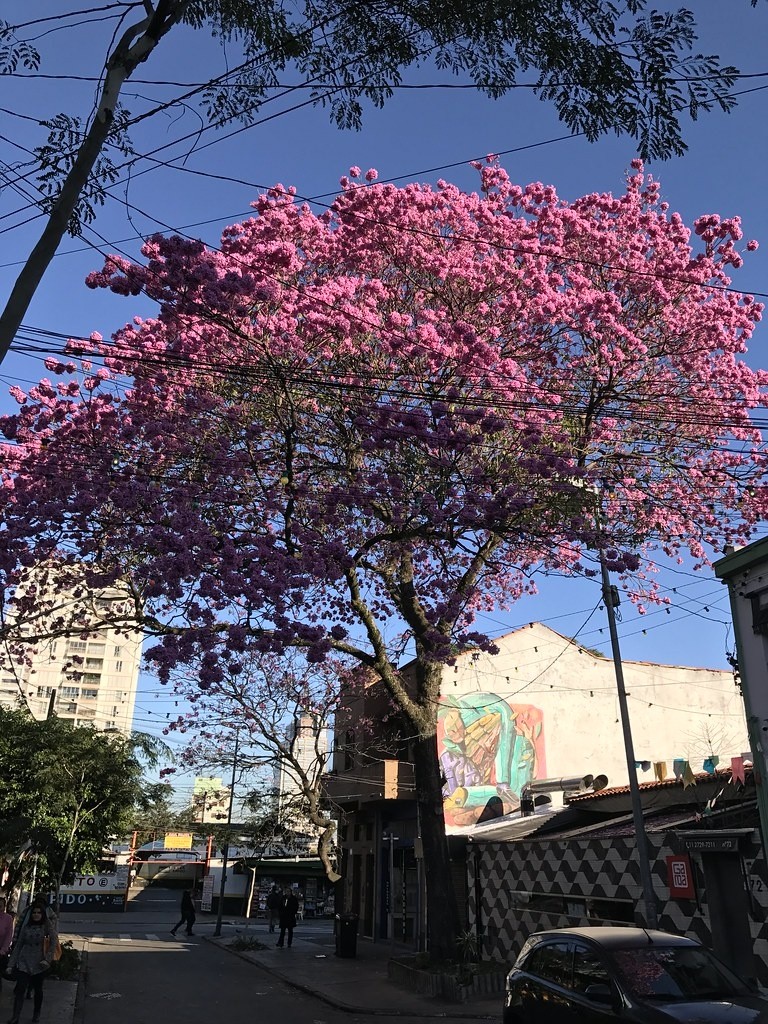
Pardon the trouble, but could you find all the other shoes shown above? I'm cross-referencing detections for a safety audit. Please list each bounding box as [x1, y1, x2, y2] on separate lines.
[275, 943, 292, 948]
[7, 1017, 19, 1024]
[188, 933, 194, 936]
[32, 1017, 39, 1022]
[169, 930, 176, 936]
[26, 991, 32, 999]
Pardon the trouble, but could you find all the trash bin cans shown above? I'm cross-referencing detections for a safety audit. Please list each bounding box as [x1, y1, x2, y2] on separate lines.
[332, 912, 360, 958]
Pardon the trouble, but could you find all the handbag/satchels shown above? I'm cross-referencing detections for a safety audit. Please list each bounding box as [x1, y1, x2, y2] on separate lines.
[44, 920, 62, 960]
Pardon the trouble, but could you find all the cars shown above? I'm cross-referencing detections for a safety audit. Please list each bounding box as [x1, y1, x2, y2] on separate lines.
[502, 926, 768, 1024]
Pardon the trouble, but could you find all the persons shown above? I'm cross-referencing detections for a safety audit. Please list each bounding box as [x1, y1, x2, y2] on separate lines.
[266, 885, 279, 934]
[170, 889, 196, 936]
[276, 888, 299, 948]
[9, 892, 60, 1000]
[0, 896, 13, 981]
[4, 903, 56, 1024]
